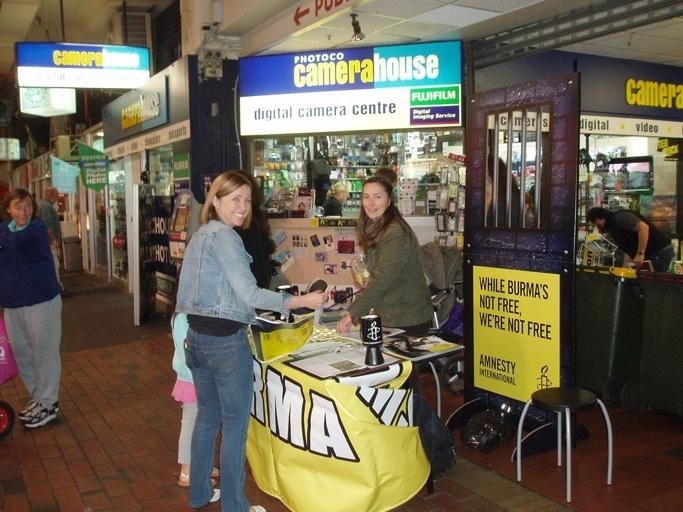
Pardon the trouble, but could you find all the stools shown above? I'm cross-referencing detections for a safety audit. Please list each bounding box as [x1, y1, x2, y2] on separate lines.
[512, 386, 616, 505]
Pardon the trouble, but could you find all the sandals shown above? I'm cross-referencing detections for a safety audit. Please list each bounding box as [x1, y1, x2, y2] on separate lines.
[177, 473, 217, 488]
[211, 468, 220, 478]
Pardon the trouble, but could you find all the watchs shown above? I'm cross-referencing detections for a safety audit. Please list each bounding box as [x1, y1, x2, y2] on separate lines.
[637, 251, 645, 257]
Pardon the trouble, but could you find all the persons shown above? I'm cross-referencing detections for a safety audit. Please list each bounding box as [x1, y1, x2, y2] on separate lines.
[173, 170, 329, 511]
[335, 177, 435, 338]
[34, 184, 75, 299]
[587, 206, 674, 273]
[170, 313, 222, 487]
[1, 187, 66, 428]
[307, 148, 537, 227]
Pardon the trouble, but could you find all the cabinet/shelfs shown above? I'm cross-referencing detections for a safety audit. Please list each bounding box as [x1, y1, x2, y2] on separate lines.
[331, 162, 397, 213]
[253, 161, 308, 216]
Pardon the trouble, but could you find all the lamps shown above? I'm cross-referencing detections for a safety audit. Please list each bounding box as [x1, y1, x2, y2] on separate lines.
[350, 13, 365, 42]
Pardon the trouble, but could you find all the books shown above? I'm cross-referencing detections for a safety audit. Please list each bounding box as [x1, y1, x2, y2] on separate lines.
[381, 333, 461, 361]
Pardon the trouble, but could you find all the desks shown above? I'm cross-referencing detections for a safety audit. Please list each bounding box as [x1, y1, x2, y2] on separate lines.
[245, 314, 463, 512]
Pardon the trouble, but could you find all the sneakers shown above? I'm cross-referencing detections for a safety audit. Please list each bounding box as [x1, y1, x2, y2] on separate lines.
[17, 401, 59, 421]
[25, 407, 57, 428]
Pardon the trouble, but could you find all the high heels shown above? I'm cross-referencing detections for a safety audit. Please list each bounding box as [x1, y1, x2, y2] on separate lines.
[251, 506, 266, 512]
[192, 488, 220, 512]
